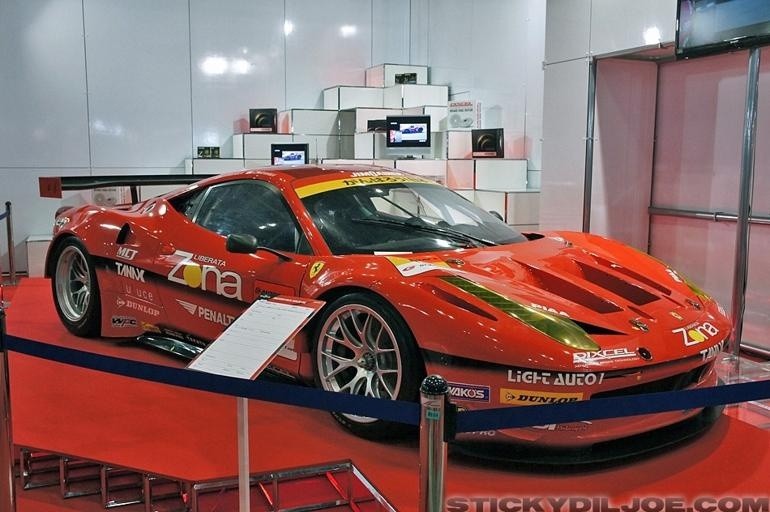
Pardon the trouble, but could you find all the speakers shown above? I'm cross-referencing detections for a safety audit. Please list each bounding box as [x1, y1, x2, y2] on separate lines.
[471, 128, 504, 158]
[249, 108, 277, 133]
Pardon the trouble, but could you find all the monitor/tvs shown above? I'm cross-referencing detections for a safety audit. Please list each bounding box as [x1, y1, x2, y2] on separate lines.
[271, 144, 309, 165]
[675, 0, 770, 62]
[386, 117, 430, 147]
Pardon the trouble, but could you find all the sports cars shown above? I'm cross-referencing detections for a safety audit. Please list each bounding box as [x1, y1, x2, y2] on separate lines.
[43, 165, 733, 468]
